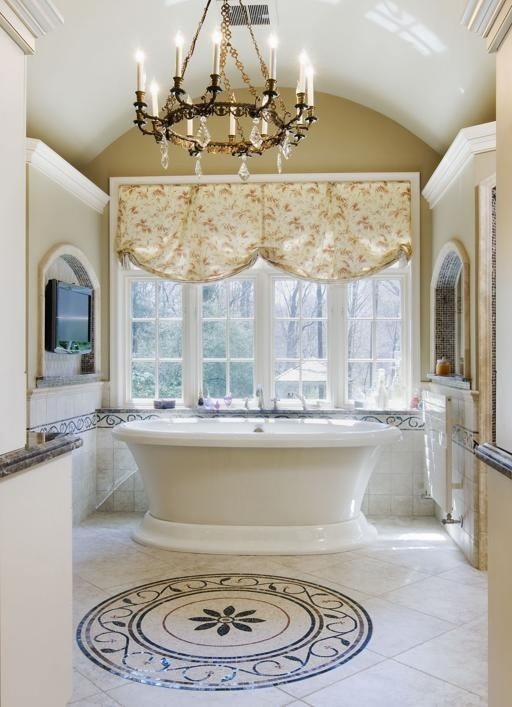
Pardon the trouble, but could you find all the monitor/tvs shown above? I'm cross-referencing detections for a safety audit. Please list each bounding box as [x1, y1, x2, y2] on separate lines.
[45, 278, 94, 355]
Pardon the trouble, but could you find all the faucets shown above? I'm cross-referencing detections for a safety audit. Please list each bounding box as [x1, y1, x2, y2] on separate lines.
[256, 384, 267, 411]
[287, 390, 308, 410]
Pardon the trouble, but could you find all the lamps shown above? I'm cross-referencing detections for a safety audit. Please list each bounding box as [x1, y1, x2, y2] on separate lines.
[133, 0, 318, 181]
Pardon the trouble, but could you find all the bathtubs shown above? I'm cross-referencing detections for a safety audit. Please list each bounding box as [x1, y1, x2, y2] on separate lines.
[111, 418, 402, 526]
